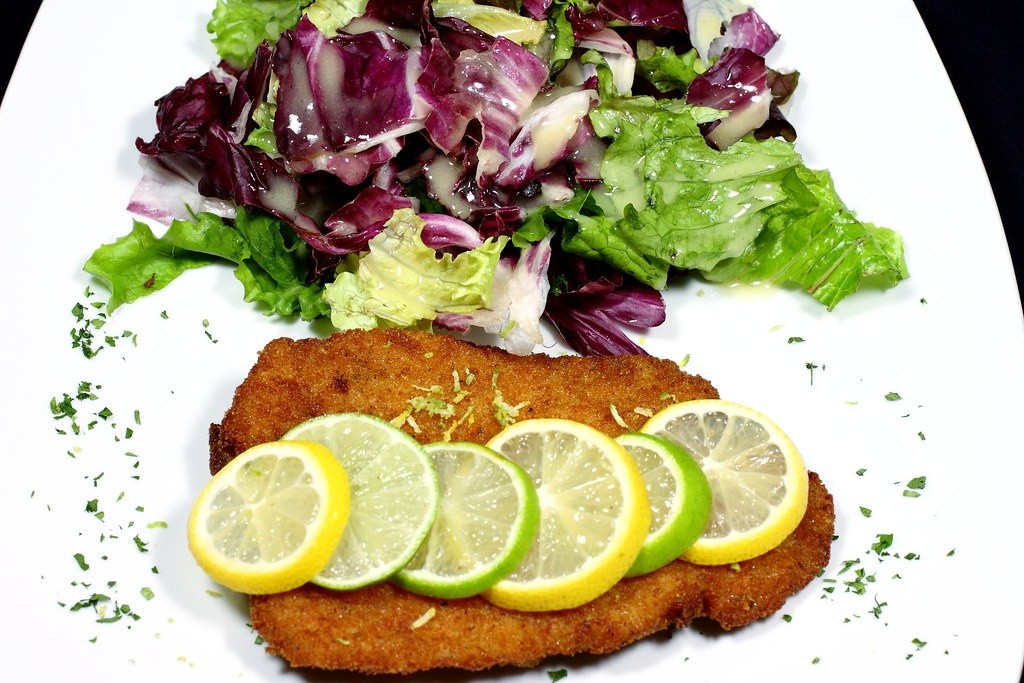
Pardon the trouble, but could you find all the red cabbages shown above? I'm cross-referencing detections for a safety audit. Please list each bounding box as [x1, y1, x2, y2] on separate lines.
[127, 0, 781, 357]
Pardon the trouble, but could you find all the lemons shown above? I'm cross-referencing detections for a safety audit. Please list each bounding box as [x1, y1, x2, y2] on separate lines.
[186, 399, 809, 614]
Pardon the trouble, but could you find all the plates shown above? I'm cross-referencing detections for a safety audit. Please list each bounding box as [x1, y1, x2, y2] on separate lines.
[0, 0, 1024, 683]
